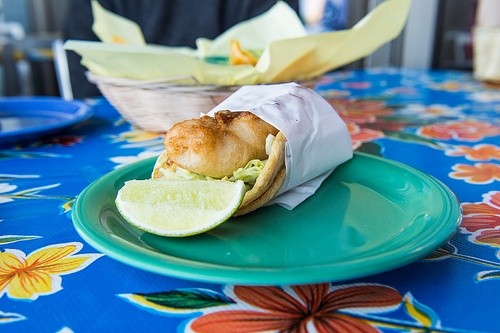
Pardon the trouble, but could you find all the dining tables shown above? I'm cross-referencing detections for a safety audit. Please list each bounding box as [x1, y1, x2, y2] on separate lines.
[0, 67, 500, 332]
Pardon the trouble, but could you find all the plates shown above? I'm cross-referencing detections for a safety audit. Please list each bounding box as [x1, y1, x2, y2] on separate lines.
[0, 97, 93, 142]
[72, 152, 463, 286]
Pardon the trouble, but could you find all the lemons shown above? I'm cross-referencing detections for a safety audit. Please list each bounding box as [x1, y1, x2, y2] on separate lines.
[115, 179, 246, 238]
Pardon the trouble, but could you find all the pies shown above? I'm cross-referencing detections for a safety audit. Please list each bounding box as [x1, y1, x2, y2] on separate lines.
[151, 110, 286, 216]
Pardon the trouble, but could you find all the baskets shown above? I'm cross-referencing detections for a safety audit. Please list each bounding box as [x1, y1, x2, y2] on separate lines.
[85, 67, 321, 133]
